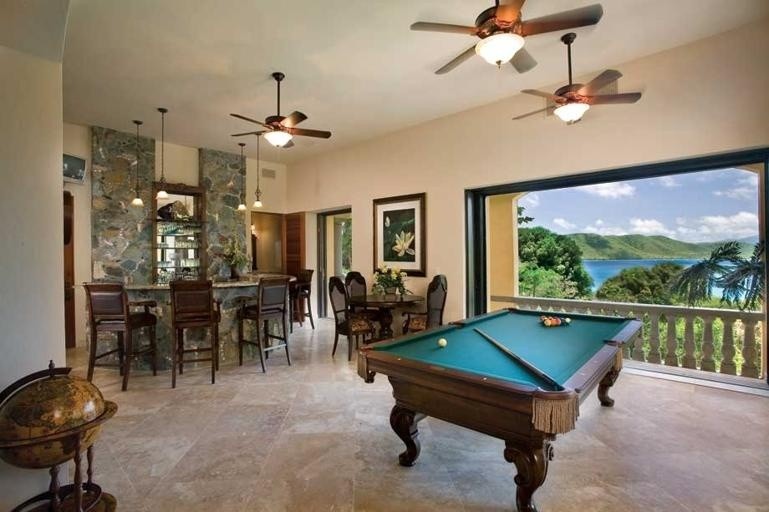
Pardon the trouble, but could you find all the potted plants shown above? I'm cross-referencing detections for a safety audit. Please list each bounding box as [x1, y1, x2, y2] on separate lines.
[216, 223, 253, 280]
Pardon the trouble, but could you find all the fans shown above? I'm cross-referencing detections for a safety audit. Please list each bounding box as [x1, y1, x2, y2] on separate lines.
[410, 0, 603, 75]
[229, 72, 332, 149]
[512, 33, 641, 121]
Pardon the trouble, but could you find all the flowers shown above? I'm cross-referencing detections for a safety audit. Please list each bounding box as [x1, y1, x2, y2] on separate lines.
[372, 262, 413, 296]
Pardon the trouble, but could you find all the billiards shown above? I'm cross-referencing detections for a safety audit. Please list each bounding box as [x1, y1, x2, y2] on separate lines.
[438, 338, 447, 347]
[541, 315, 571, 326]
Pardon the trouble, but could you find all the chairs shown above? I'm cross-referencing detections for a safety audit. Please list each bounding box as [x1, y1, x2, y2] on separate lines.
[81, 283, 157, 391]
[233, 276, 291, 374]
[329, 272, 448, 361]
[168, 279, 221, 388]
[289, 269, 315, 333]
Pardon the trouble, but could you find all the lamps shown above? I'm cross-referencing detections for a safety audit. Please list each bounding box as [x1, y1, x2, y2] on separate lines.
[553, 103, 590, 125]
[156, 107, 169, 199]
[475, 33, 525, 72]
[263, 131, 293, 148]
[237, 141, 246, 211]
[252, 132, 264, 208]
[130, 119, 143, 208]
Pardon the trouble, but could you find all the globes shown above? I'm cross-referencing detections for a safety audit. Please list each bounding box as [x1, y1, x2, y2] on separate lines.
[0, 360, 117, 512]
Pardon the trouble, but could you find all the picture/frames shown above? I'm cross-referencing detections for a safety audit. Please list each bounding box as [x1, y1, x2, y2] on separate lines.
[373, 192, 426, 277]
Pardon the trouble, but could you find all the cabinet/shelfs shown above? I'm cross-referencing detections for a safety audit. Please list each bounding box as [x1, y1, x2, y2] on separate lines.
[155, 218, 202, 286]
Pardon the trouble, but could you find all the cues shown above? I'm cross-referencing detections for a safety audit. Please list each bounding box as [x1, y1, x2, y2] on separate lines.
[474, 327, 558, 387]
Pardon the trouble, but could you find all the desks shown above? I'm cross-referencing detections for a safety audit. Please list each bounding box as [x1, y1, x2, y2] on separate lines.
[357, 306, 645, 512]
